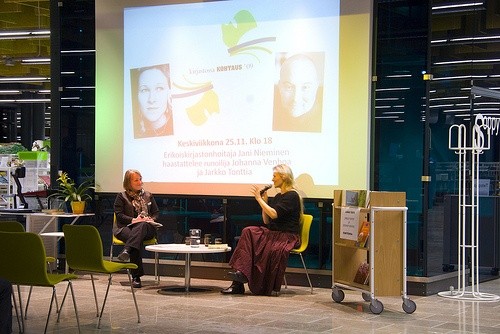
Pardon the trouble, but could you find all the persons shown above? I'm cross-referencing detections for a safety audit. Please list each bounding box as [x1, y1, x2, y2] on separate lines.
[272, 54, 322, 130]
[113, 169, 158, 288]
[221, 165, 304, 295]
[135, 68, 170, 137]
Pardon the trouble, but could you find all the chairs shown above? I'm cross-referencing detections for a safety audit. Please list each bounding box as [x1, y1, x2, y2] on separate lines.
[0, 213, 160, 334]
[284, 214, 314, 293]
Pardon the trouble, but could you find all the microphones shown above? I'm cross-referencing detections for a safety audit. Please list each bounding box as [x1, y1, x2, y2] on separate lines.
[259, 184, 273, 195]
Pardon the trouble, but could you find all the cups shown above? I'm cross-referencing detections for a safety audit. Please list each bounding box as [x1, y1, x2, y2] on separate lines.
[189, 229, 201, 248]
[185, 237, 190, 245]
[204, 234, 211, 246]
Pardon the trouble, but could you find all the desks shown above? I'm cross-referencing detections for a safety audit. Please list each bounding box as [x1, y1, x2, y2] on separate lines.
[145, 244, 232, 295]
[0, 212, 95, 270]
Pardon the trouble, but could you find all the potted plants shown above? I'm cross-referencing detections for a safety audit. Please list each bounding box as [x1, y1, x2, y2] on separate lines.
[46, 171, 96, 214]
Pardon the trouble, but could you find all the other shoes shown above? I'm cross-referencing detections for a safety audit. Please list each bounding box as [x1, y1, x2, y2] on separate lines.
[220, 286, 245, 295]
[227, 271, 248, 283]
[132, 277, 141, 288]
[118, 249, 130, 260]
